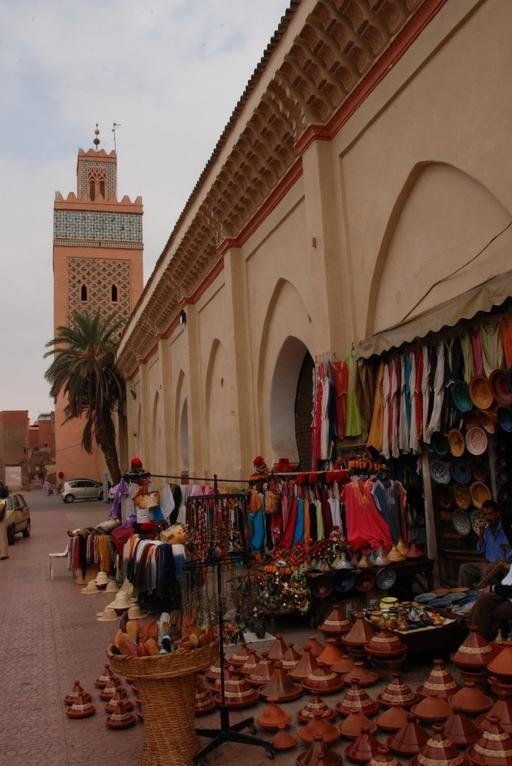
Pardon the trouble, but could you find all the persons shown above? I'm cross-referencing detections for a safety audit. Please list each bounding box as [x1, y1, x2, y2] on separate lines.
[458, 500, 512, 590]
[0, 480, 9, 560]
[249, 455, 272, 494]
[131, 458, 153, 493]
[482, 560, 512, 599]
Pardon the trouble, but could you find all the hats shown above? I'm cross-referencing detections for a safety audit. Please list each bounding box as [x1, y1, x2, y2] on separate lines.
[79, 570, 119, 595]
[95, 590, 148, 623]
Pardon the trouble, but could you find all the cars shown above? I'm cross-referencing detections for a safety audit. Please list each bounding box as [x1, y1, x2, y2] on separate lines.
[60, 477, 104, 503]
[5, 493, 31, 545]
